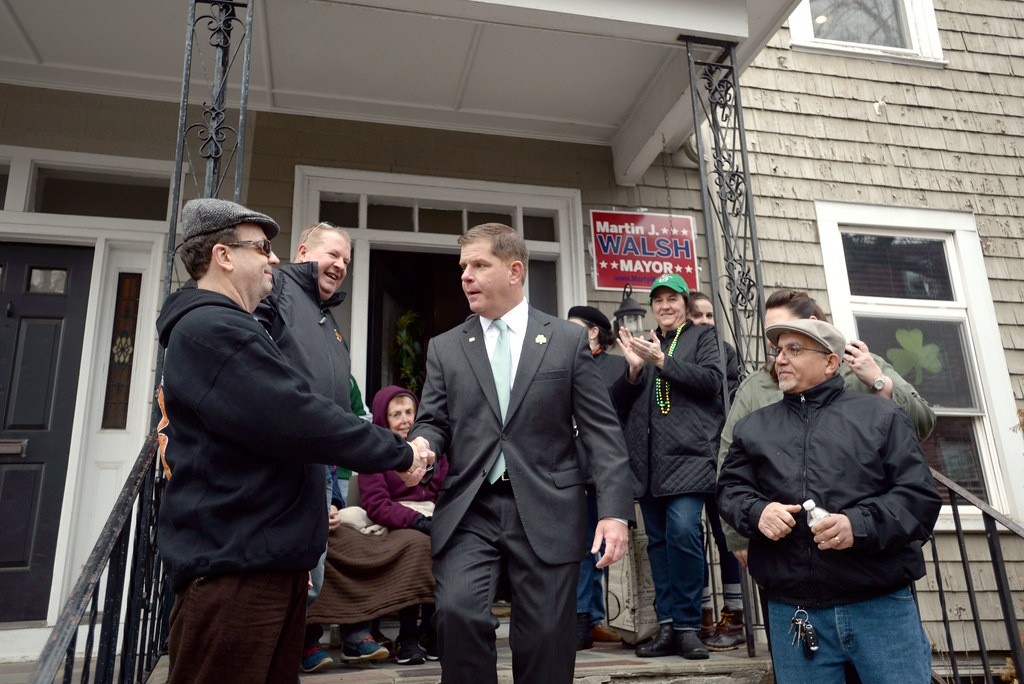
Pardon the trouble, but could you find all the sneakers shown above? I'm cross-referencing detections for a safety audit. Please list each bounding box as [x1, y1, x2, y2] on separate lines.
[394, 636, 426, 665]
[301, 644, 333, 672]
[417, 631, 440, 661]
[340, 636, 389, 661]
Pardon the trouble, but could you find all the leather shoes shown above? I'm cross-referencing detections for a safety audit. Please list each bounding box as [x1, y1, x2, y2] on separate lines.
[590, 624, 621, 642]
[634, 624, 676, 657]
[676, 630, 710, 659]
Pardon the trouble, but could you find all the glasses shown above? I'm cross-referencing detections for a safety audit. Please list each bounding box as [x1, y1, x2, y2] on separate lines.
[772, 345, 831, 358]
[389, 410, 415, 419]
[303, 222, 333, 244]
[222, 240, 272, 257]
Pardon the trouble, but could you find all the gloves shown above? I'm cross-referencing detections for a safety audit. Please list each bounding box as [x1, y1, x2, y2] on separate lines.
[412, 513, 434, 536]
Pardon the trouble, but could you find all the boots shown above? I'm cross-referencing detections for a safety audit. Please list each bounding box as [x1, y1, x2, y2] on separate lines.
[575, 612, 592, 651]
[704, 605, 747, 652]
[698, 607, 717, 642]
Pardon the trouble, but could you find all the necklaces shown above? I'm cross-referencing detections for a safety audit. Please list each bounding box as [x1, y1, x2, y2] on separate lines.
[654, 321, 687, 414]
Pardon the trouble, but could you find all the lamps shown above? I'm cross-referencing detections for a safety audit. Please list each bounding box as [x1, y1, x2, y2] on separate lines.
[611, 283, 646, 337]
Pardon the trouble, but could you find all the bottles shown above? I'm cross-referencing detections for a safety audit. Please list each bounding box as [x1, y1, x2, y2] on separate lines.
[803, 499, 831, 544]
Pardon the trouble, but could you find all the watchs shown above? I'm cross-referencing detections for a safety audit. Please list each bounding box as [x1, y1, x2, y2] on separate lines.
[867, 374, 887, 392]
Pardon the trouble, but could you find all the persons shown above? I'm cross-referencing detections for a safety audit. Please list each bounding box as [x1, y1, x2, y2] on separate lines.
[715, 318, 943, 684]
[717, 288, 936, 571]
[398, 224, 629, 683]
[298, 465, 388, 673]
[252, 219, 367, 597]
[565, 306, 632, 648]
[684, 289, 746, 651]
[606, 271, 726, 658]
[355, 383, 440, 665]
[153, 198, 433, 684]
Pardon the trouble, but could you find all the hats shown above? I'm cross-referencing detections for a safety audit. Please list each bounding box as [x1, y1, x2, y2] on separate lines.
[649, 274, 689, 296]
[568, 306, 612, 332]
[765, 319, 846, 367]
[183, 198, 281, 242]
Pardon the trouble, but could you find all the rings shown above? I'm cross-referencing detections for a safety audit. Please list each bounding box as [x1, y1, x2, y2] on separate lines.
[835, 536, 841, 544]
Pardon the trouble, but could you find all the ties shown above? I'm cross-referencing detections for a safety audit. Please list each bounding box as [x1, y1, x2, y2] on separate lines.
[485, 319, 511, 485]
